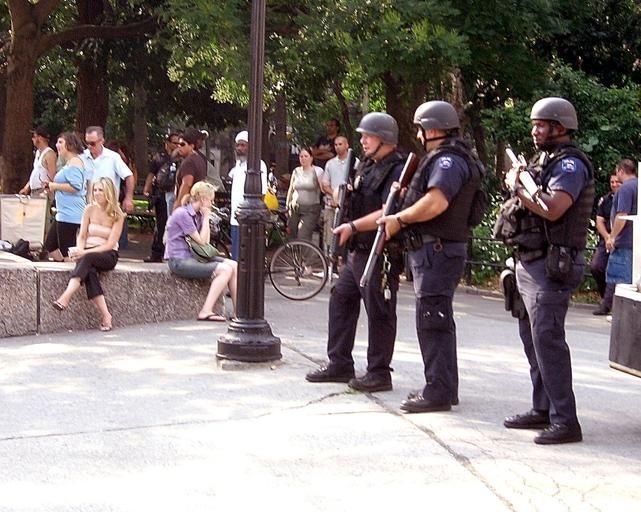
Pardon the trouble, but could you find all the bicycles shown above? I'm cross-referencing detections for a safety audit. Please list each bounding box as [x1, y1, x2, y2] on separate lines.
[208, 205, 328, 300]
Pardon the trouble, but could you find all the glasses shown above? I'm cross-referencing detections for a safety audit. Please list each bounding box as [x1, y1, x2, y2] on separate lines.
[169, 141, 178, 144]
[86, 140, 101, 146]
[180, 143, 186, 146]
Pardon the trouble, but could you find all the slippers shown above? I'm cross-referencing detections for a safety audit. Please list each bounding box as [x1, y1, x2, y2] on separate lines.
[198, 313, 225, 321]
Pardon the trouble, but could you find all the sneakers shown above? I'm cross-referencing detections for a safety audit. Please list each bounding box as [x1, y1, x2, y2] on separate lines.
[143, 256, 163, 262]
[535, 422, 581, 444]
[348, 371, 392, 391]
[52, 300, 66, 311]
[592, 305, 609, 313]
[504, 410, 550, 428]
[401, 392, 458, 410]
[100, 324, 111, 331]
[305, 365, 355, 382]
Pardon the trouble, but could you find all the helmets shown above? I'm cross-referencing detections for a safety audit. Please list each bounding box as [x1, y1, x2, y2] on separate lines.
[356, 112, 399, 145]
[414, 101, 460, 130]
[530, 97, 578, 130]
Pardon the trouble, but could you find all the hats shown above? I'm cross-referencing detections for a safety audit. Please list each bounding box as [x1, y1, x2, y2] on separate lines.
[29, 127, 51, 138]
[235, 131, 249, 143]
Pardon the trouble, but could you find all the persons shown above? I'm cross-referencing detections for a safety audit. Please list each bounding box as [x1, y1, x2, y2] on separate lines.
[493, 98, 595, 445]
[286, 117, 361, 276]
[306, 112, 407, 393]
[19, 127, 138, 262]
[51, 177, 126, 331]
[376, 101, 479, 413]
[163, 182, 237, 322]
[590, 159, 638, 314]
[142, 128, 267, 282]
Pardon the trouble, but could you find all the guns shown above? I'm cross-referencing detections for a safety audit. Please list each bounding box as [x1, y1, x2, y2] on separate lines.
[360, 152, 421, 289]
[328, 148, 357, 286]
[505, 147, 549, 212]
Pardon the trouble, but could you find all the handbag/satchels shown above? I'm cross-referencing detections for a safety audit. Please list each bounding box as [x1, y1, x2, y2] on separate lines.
[271, 224, 297, 242]
[14, 239, 29, 257]
[186, 235, 218, 263]
[264, 189, 278, 210]
